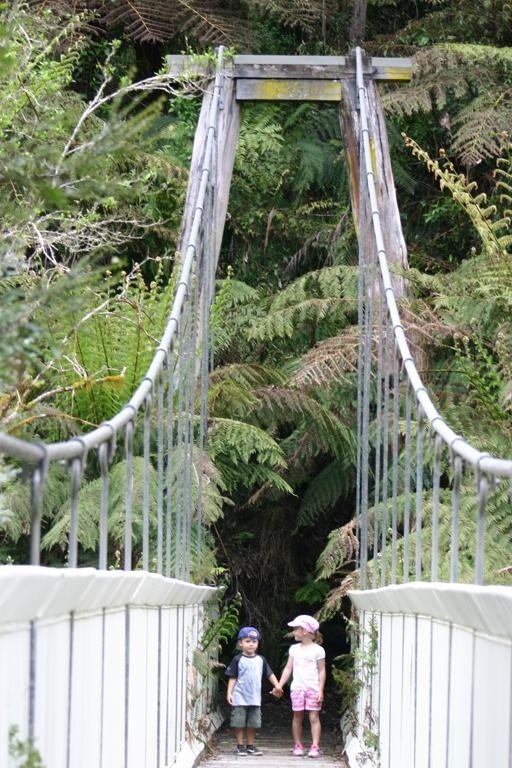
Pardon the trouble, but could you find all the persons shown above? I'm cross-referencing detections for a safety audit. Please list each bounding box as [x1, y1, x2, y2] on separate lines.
[224, 615, 326, 759]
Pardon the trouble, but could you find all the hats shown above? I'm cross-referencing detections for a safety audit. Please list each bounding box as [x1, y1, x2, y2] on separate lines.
[237, 626, 260, 641]
[286, 614, 320, 634]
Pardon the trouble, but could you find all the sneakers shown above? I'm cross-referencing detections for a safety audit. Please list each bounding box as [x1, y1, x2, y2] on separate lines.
[307, 744, 322, 758]
[235, 745, 264, 757]
[293, 742, 305, 757]
